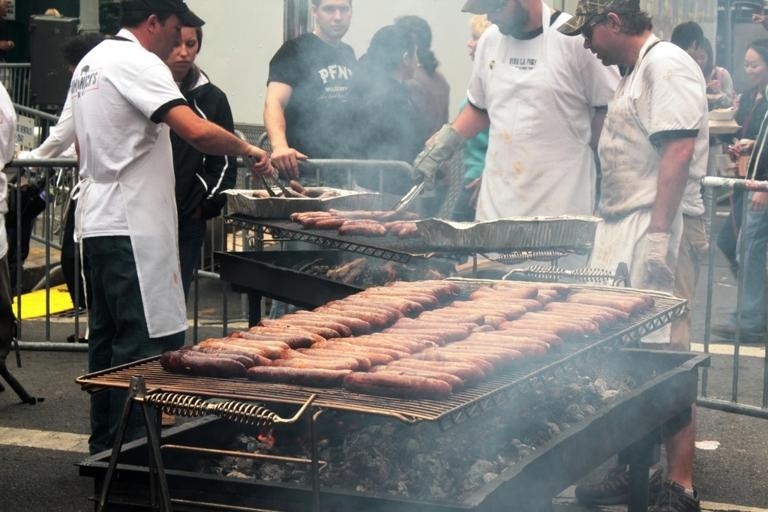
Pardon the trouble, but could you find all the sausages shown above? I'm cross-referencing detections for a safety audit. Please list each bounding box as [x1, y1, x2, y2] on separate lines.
[248, 180, 342, 199]
[342, 289, 654, 395]
[245, 282, 568, 386]
[289, 208, 424, 238]
[161, 279, 461, 375]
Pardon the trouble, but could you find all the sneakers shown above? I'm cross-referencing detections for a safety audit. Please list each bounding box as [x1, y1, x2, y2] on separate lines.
[574, 463, 664, 505]
[648, 478, 702, 512]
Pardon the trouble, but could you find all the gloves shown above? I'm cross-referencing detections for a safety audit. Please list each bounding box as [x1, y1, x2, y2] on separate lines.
[644, 232, 674, 283]
[411, 123, 466, 195]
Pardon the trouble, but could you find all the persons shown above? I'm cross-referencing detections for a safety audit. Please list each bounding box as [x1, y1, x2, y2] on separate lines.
[670, 11, 767, 343]
[393, 14, 449, 142]
[346, 23, 426, 197]
[163, 25, 238, 305]
[262, 0, 357, 319]
[449, 12, 494, 222]
[554, 0, 710, 512]
[409, 0, 623, 274]
[0, 0, 126, 393]
[68, 0, 274, 458]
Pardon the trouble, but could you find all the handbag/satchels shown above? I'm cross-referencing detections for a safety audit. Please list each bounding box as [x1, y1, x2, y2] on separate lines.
[738, 154, 752, 177]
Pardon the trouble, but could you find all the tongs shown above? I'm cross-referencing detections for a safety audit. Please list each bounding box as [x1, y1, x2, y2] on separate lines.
[95, 373, 173, 511]
[390, 173, 425, 214]
[241, 152, 295, 198]
[612, 261, 632, 288]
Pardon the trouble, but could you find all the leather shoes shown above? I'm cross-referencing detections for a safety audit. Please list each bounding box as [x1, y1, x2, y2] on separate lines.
[706, 321, 766, 344]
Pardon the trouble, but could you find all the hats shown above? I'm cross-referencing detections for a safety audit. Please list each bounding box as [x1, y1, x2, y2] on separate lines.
[556, 0, 639, 36]
[119, 0, 206, 27]
[461, 0, 507, 14]
